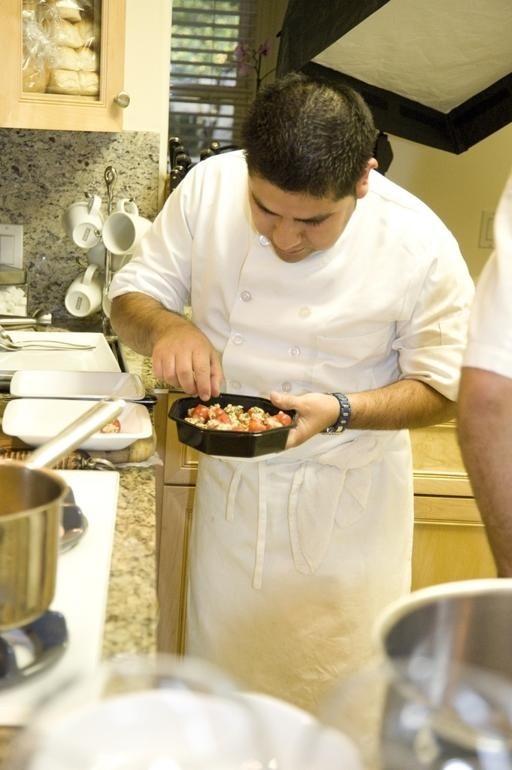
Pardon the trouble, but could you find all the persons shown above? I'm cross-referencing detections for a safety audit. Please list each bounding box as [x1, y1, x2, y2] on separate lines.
[453, 172, 511, 580]
[105, 72, 474, 769]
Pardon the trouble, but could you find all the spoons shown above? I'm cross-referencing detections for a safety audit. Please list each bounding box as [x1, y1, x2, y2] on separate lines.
[0, 326, 96, 352]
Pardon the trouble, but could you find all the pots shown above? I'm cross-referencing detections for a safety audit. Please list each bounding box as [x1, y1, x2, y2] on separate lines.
[376, 574, 512, 770]
[0, 453, 89, 688]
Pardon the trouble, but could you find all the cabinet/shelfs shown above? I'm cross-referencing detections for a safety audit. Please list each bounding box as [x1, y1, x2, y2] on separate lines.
[0, 0, 131, 135]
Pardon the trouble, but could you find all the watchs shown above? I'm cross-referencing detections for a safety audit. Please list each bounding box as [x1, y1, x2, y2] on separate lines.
[320, 392, 352, 435]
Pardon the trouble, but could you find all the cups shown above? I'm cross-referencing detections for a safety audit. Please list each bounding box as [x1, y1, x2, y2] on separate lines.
[305, 652, 512, 770]
[19, 648, 273, 770]
[105, 198, 154, 255]
[64, 265, 104, 316]
[87, 244, 127, 270]
[58, 196, 103, 249]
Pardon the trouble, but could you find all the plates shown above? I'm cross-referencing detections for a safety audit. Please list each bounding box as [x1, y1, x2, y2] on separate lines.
[4, 397, 153, 453]
[8, 367, 146, 401]
[169, 390, 304, 455]
